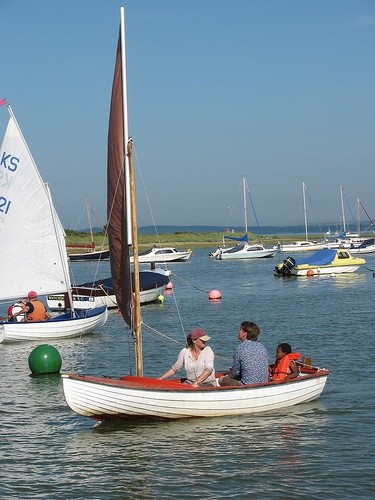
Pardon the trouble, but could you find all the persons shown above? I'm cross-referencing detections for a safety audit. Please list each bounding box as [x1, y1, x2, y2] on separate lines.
[219, 321, 269, 387]
[217, 246, 222, 254]
[8, 291, 51, 323]
[268, 343, 299, 382]
[157, 328, 217, 389]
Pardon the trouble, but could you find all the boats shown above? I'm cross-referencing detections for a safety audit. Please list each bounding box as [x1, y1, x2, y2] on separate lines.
[49, 262, 170, 304]
[209, 246, 231, 258]
[276, 247, 366, 276]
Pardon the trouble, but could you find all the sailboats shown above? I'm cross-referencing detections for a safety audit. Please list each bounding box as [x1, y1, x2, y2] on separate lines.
[324, 186, 360, 238]
[59, 8, 327, 419]
[324, 198, 375, 253]
[217, 177, 279, 259]
[273, 181, 326, 251]
[0, 104, 109, 343]
[131, 246, 193, 263]
[69, 194, 109, 259]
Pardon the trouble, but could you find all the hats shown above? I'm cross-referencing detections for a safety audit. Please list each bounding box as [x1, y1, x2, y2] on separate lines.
[28, 291, 37, 300]
[20, 298, 27, 305]
[190, 328, 211, 342]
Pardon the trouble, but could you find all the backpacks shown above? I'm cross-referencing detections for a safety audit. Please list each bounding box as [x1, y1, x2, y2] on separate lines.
[8, 304, 23, 322]
